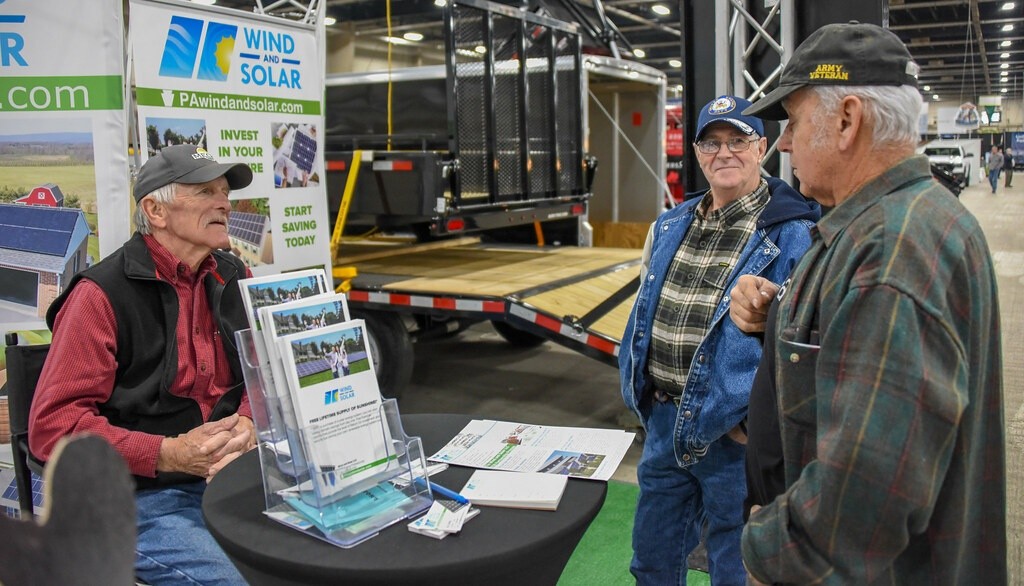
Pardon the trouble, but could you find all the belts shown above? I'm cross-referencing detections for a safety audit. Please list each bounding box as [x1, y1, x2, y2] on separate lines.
[650, 385, 674, 403]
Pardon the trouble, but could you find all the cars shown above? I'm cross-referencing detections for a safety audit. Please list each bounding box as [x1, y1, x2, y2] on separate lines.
[1013, 139, 1024, 170]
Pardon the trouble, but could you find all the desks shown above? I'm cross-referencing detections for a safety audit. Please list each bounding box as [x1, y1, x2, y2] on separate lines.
[201, 414, 608, 586]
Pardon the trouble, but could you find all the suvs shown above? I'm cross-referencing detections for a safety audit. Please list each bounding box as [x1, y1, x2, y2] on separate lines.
[922, 143, 973, 190]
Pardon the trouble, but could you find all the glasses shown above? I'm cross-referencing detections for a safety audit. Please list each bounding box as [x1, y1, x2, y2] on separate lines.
[694, 136, 757, 155]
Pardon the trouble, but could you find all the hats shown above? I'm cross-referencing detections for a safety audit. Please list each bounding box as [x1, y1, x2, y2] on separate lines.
[740, 20, 921, 121]
[695, 95, 764, 142]
[134, 144, 254, 205]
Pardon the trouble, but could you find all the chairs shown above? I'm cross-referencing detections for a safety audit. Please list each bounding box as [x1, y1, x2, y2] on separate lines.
[5, 332, 46, 519]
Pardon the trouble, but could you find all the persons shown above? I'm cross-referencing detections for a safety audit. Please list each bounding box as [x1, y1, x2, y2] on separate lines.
[28, 144, 268, 586]
[279, 284, 302, 303]
[618, 93, 821, 586]
[323, 338, 350, 378]
[303, 309, 326, 329]
[729, 20, 1015, 586]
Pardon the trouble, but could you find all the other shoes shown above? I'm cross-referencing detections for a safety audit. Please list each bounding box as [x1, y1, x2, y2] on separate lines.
[992, 190, 995, 194]
[1005, 185, 1013, 187]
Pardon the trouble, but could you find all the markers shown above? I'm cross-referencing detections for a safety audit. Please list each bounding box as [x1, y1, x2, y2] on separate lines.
[415, 477, 468, 505]
